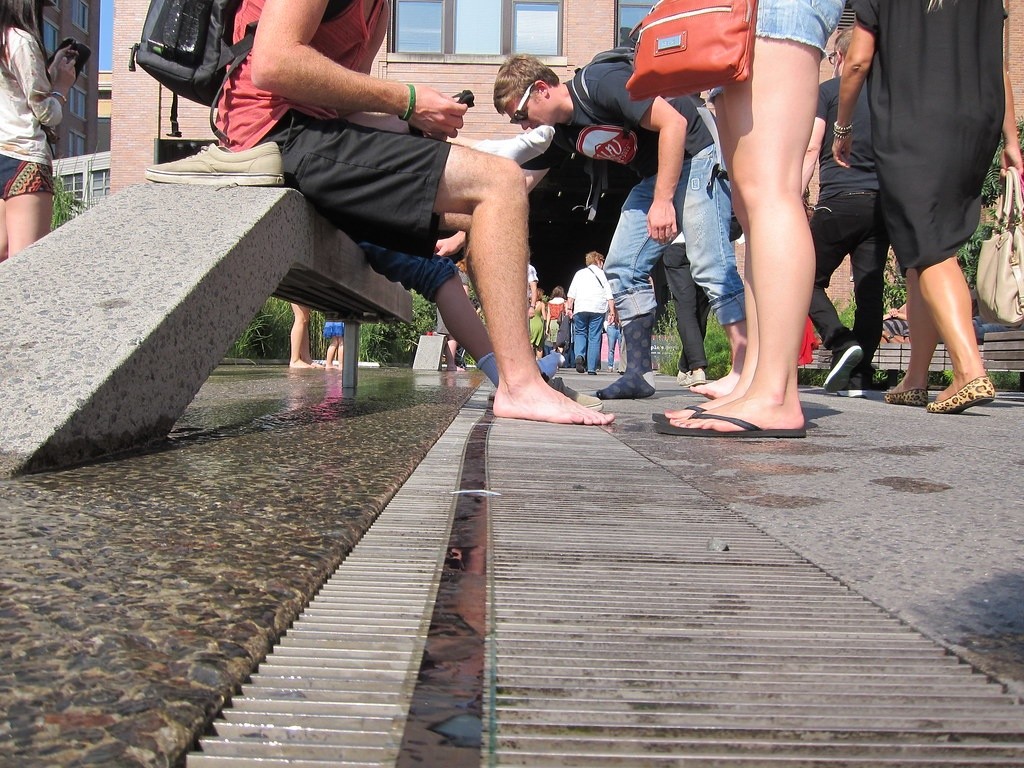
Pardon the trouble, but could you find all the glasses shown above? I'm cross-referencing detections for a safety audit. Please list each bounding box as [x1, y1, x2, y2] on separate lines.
[829, 52, 838, 65]
[509, 82, 537, 124]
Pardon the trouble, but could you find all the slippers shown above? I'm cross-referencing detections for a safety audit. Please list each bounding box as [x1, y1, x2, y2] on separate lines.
[654, 414, 806, 438]
[652, 406, 708, 422]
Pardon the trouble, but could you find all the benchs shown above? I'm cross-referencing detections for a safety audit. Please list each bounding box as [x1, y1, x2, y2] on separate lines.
[0, 179, 447, 481]
[798, 332, 1024, 387]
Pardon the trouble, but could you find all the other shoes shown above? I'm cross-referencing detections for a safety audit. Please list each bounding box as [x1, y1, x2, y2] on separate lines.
[607, 368, 613, 372]
[620, 372, 624, 374]
[848, 383, 871, 398]
[822, 345, 864, 392]
[589, 372, 596, 375]
[884, 389, 928, 406]
[576, 355, 585, 373]
[926, 376, 995, 415]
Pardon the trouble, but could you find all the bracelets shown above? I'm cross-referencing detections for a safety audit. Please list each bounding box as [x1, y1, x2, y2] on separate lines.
[530, 306, 535, 309]
[399, 83, 415, 122]
[610, 314, 615, 317]
[567, 307, 572, 310]
[52, 91, 67, 103]
[832, 120, 853, 139]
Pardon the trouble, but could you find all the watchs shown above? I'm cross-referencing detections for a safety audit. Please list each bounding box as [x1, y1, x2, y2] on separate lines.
[887, 334, 894, 341]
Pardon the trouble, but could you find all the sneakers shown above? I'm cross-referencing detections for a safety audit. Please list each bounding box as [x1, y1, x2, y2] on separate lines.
[548, 377, 603, 412]
[679, 368, 707, 387]
[676, 371, 692, 384]
[145, 141, 285, 187]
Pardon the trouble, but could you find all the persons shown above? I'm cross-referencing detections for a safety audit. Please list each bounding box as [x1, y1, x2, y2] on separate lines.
[0, 0, 79, 262]
[434, 47, 750, 400]
[650, 1, 845, 437]
[830, 0, 1024, 413]
[289, 301, 323, 370]
[323, 322, 344, 370]
[800, 24, 890, 398]
[879, 303, 1020, 344]
[352, 124, 564, 389]
[217, 0, 615, 425]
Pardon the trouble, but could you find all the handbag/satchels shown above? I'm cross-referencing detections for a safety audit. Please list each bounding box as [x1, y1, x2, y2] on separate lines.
[625, 0, 758, 100]
[976, 166, 1024, 327]
[556, 315, 571, 355]
[557, 301, 565, 326]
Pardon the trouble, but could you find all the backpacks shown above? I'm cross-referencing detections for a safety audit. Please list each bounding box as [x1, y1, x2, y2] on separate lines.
[129, 0, 355, 148]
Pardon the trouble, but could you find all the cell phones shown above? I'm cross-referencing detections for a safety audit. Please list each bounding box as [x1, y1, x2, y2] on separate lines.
[65, 50, 79, 64]
